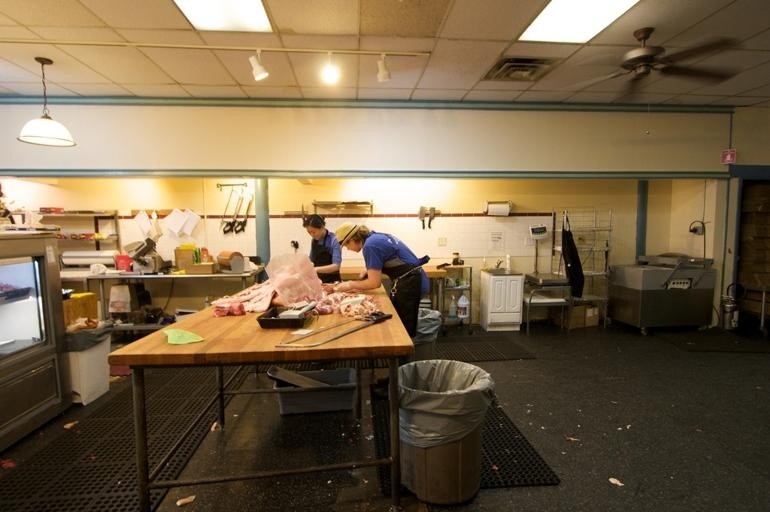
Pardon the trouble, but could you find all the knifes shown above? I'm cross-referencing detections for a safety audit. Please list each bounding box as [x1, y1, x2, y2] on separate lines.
[419, 207, 425, 229]
[428, 208, 435, 228]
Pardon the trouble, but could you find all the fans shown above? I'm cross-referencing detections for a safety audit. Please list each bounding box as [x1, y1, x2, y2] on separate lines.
[556, 24, 742, 99]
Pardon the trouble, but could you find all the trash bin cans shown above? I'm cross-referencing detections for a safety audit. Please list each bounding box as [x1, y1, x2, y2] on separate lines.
[412, 308, 441, 360]
[65, 320, 115, 405]
[398, 360, 495, 504]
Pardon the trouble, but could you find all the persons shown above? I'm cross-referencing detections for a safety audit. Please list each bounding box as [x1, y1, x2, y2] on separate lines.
[302, 214, 342, 283]
[331, 221, 431, 366]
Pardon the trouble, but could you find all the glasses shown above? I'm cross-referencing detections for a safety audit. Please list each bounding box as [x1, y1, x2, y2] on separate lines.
[303, 216, 318, 221]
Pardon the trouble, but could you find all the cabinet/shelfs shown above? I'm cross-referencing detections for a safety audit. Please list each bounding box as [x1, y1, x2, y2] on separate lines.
[8, 210, 120, 298]
[479, 269, 524, 331]
[552, 206, 613, 333]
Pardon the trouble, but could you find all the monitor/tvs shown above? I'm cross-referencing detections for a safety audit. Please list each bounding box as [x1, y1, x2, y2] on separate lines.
[0, 251, 52, 365]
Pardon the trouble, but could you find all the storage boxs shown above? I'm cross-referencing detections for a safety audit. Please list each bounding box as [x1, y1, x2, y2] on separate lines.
[564, 305, 599, 330]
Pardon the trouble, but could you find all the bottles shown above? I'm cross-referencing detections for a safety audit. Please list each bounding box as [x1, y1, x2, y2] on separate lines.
[449, 294, 457, 316]
[453, 253, 459, 265]
[194, 248, 200, 263]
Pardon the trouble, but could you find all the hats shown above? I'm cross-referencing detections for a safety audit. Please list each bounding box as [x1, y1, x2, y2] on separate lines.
[335, 223, 360, 247]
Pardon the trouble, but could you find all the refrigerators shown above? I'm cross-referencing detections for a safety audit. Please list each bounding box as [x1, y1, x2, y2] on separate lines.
[0, 231, 74, 451]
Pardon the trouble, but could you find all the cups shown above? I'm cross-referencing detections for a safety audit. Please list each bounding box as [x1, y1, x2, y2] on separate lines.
[460, 260, 464, 264]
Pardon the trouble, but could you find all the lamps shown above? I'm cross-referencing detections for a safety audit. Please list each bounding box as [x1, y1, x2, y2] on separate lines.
[14, 56, 74, 148]
[375, 53, 393, 82]
[318, 52, 342, 86]
[246, 48, 274, 82]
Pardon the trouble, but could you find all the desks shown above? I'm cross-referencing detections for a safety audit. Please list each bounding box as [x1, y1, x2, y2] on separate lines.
[83, 266, 263, 336]
[340, 265, 446, 313]
[105, 282, 415, 512]
